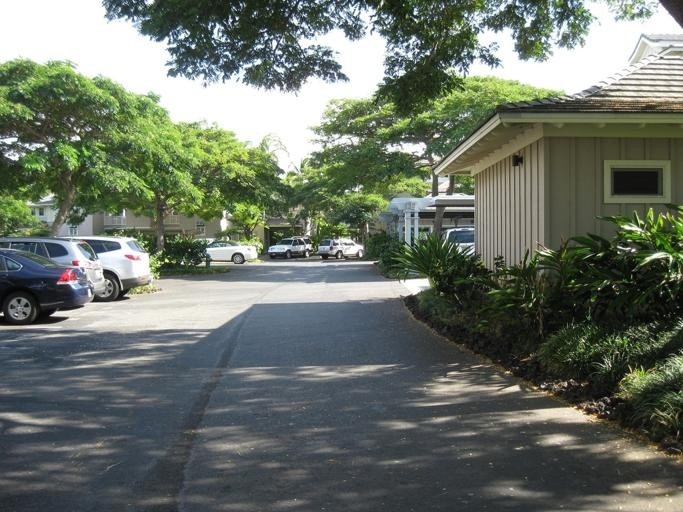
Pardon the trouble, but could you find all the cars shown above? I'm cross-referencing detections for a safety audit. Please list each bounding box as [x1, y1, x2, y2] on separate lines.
[267, 236, 363, 259]
[0, 235, 152, 325]
[191, 241, 257, 265]
[440, 227, 474, 255]
